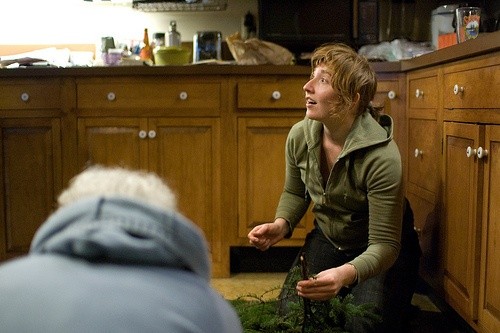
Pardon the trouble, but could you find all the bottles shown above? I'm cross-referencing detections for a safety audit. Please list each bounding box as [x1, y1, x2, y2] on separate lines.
[140, 28, 155, 65]
[241, 10, 256, 40]
[165, 20, 180, 47]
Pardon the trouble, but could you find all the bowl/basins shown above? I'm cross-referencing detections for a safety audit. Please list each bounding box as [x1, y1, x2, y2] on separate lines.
[152, 46, 191, 65]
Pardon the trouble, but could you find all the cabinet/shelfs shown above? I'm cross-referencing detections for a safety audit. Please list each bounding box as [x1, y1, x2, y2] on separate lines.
[0, 49, 500, 333]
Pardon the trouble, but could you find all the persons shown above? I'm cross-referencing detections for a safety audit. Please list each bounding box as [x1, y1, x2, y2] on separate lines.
[0, 165, 243, 333]
[247, 43, 420, 333]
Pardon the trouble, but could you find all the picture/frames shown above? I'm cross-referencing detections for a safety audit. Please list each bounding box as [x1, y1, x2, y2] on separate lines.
[193, 31, 222, 62]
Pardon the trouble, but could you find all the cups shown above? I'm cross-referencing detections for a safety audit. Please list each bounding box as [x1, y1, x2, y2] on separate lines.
[456, 7, 481, 43]
[108, 48, 123, 66]
[102, 37, 115, 66]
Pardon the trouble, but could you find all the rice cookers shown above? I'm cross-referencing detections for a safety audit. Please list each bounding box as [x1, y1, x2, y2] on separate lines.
[431, 4, 460, 50]
